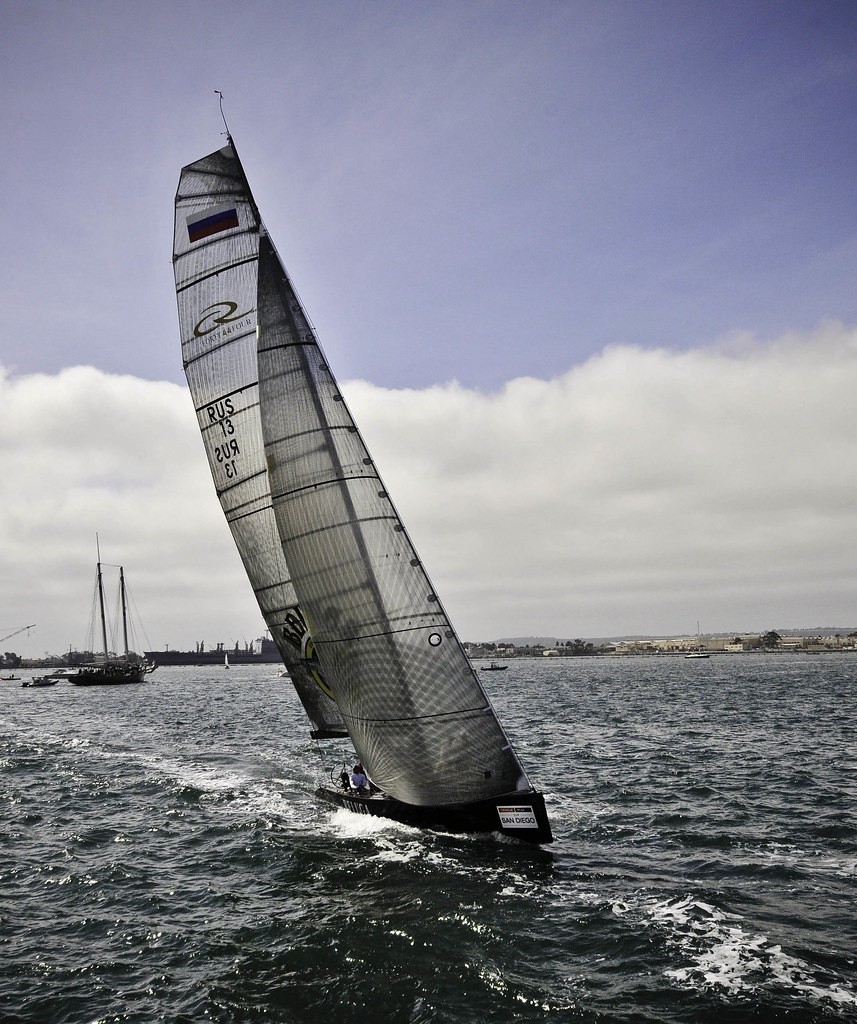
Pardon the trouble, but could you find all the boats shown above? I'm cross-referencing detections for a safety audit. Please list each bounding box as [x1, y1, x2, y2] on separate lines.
[1, 674, 21, 680]
[44, 669, 79, 678]
[278, 666, 290, 677]
[480, 660, 508, 671]
[20, 676, 59, 687]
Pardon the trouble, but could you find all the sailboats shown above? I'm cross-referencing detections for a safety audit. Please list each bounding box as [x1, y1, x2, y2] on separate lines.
[108, 566, 158, 675]
[172, 90, 554, 848]
[225, 653, 230, 669]
[685, 620, 709, 658]
[68, 644, 76, 670]
[67, 532, 144, 686]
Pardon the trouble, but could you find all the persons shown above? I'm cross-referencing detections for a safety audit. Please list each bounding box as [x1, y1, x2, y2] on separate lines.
[79, 667, 127, 676]
[357, 762, 367, 779]
[352, 766, 366, 788]
